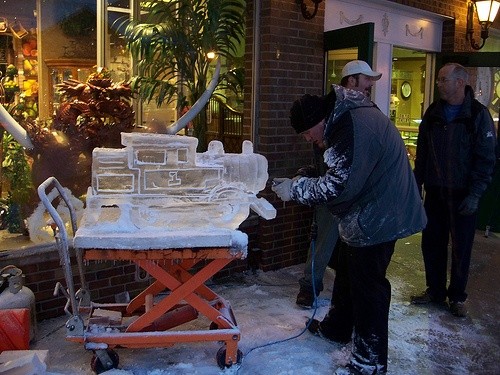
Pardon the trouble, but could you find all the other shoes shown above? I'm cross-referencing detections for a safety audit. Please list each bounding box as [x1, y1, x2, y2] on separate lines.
[296, 288, 315, 308]
[410, 291, 446, 303]
[449, 300, 468, 316]
[306, 318, 321, 336]
[335, 362, 369, 375]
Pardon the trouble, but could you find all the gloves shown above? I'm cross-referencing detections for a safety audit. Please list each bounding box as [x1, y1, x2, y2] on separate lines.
[458, 186, 482, 215]
[414, 174, 424, 200]
[272, 178, 293, 202]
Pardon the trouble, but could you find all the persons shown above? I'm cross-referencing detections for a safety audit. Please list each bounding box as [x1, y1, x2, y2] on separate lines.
[296, 61, 383, 306]
[271, 83, 427, 375]
[410, 62, 497, 317]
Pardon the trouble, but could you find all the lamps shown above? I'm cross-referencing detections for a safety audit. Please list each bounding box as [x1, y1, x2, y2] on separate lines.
[466, 0, 500, 49]
[295, 0, 322, 19]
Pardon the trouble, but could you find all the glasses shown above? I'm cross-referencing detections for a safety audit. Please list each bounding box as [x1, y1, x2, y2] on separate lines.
[436, 78, 457, 84]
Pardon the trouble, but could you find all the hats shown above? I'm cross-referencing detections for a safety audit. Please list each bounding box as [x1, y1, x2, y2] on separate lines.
[290, 94, 331, 134]
[343, 60, 382, 81]
